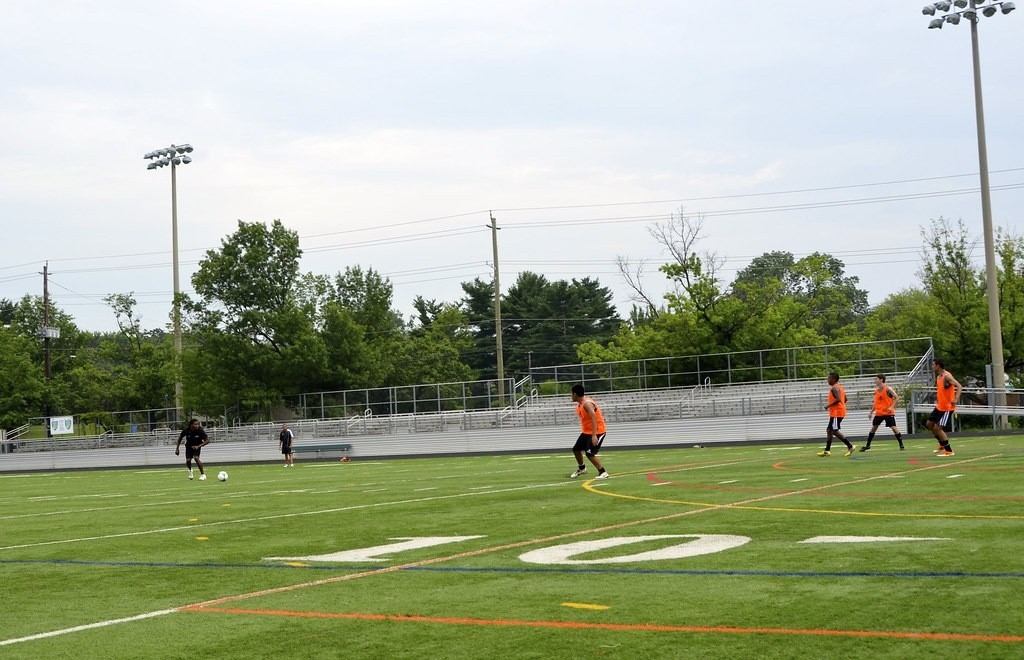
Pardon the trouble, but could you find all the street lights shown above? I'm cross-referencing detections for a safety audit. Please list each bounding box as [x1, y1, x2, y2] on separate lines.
[142, 142, 195, 431]
[920, 0, 1018, 432]
[494, 323, 522, 407]
[524, 351, 535, 396]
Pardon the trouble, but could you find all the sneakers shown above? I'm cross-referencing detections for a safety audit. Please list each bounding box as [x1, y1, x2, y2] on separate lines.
[817, 450, 830, 456]
[595, 472, 609, 479]
[188, 468, 193, 479]
[199, 474, 206, 480]
[933, 445, 944, 453]
[845, 445, 856, 456]
[937, 451, 954, 456]
[571, 468, 587, 478]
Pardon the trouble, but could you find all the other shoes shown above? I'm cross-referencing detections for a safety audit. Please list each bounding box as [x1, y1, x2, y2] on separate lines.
[284, 464, 288, 467]
[899, 445, 904, 450]
[291, 464, 294, 467]
[860, 446, 870, 452]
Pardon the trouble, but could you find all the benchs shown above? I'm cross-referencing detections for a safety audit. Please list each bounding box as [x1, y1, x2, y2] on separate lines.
[13, 373, 932, 463]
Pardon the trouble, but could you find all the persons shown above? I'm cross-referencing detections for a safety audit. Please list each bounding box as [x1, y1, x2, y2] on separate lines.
[175, 418, 210, 481]
[279, 423, 294, 467]
[570, 383, 610, 479]
[816, 372, 856, 457]
[926, 358, 962, 457]
[859, 373, 905, 452]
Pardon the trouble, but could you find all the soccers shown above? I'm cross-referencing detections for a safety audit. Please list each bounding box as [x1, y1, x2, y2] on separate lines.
[217, 471, 228, 482]
